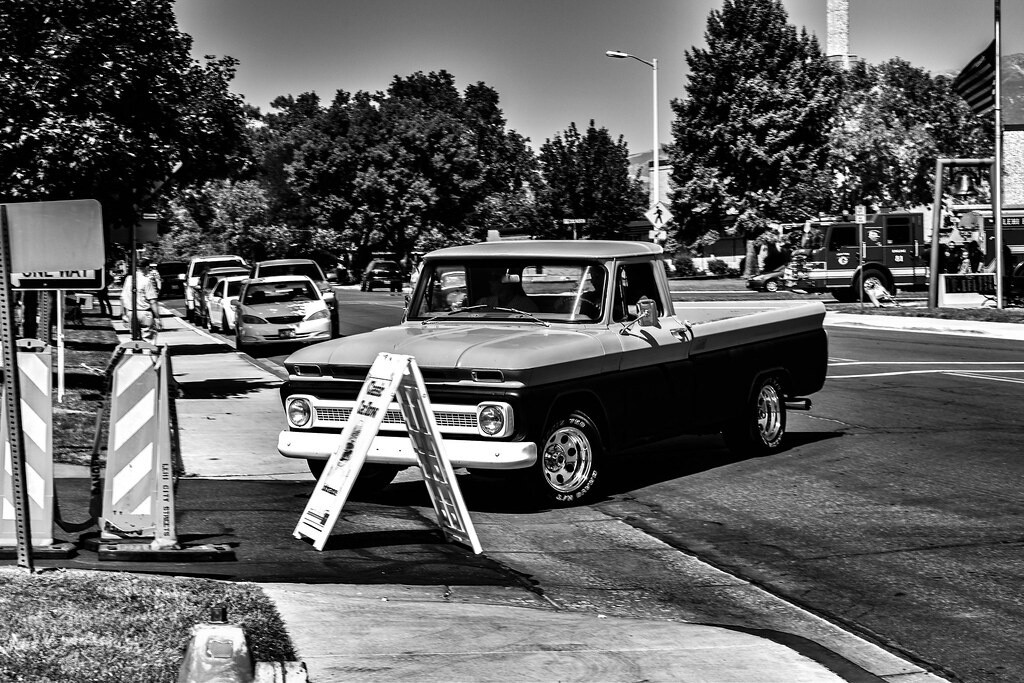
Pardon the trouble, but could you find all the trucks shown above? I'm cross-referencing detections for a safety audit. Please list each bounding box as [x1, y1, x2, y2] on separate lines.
[798, 203, 1024, 302]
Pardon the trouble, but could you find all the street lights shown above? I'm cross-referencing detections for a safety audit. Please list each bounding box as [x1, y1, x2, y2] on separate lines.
[604, 48, 662, 244]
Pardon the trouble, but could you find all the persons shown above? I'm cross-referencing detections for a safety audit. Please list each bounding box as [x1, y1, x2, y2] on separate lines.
[97, 273, 114, 317]
[116, 258, 162, 345]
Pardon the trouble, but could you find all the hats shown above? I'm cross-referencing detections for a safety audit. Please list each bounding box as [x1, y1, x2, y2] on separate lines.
[136, 258, 153, 267]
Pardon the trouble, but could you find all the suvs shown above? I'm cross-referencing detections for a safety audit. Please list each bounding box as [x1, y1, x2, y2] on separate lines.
[257, 259, 339, 340]
[177, 255, 244, 324]
[154, 260, 187, 303]
[190, 266, 250, 329]
[361, 260, 404, 292]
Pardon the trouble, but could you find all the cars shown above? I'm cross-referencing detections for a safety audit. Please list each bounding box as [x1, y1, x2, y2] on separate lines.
[406, 259, 467, 319]
[203, 276, 250, 335]
[231, 273, 335, 355]
[745, 265, 786, 293]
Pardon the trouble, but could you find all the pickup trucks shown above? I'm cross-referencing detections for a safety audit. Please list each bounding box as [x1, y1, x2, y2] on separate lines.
[276, 241, 830, 510]
